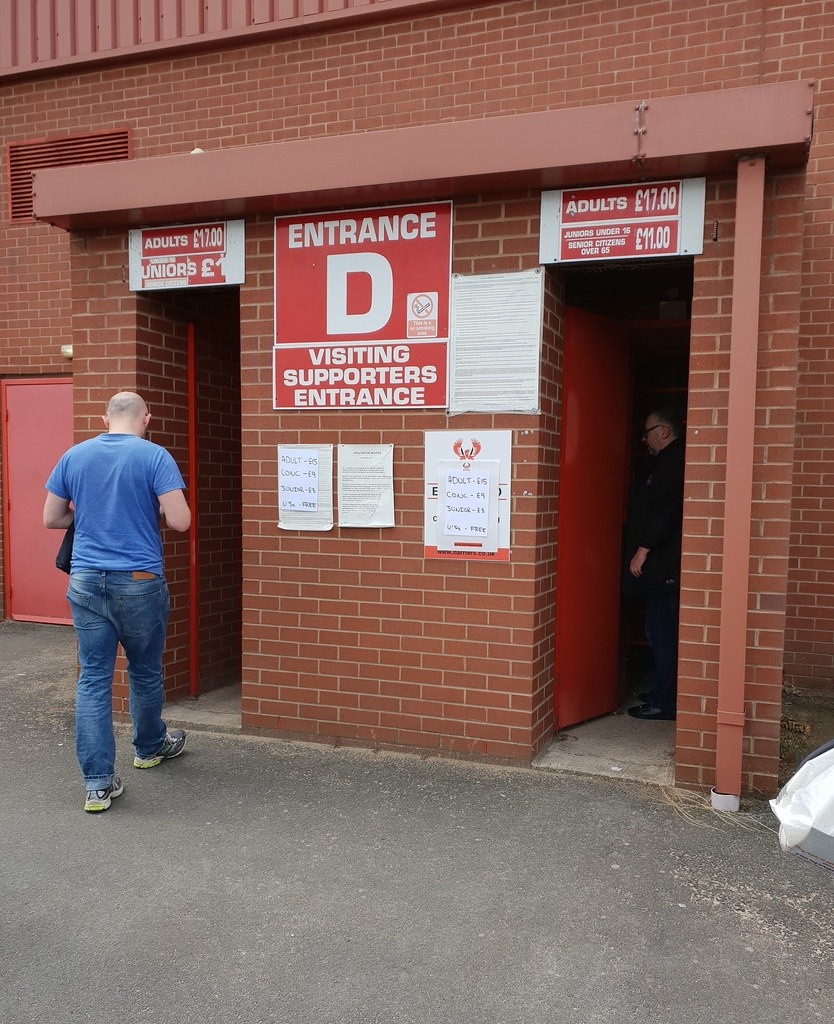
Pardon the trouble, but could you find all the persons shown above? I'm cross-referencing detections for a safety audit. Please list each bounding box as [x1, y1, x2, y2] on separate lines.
[626, 406, 685, 720]
[43, 392, 193, 811]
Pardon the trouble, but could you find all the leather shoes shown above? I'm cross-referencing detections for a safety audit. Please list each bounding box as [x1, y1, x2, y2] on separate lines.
[628, 702, 677, 720]
[636, 693, 653, 701]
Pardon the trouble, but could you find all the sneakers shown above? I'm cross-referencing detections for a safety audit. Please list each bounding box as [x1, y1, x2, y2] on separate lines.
[84, 777, 123, 811]
[133, 730, 186, 768]
